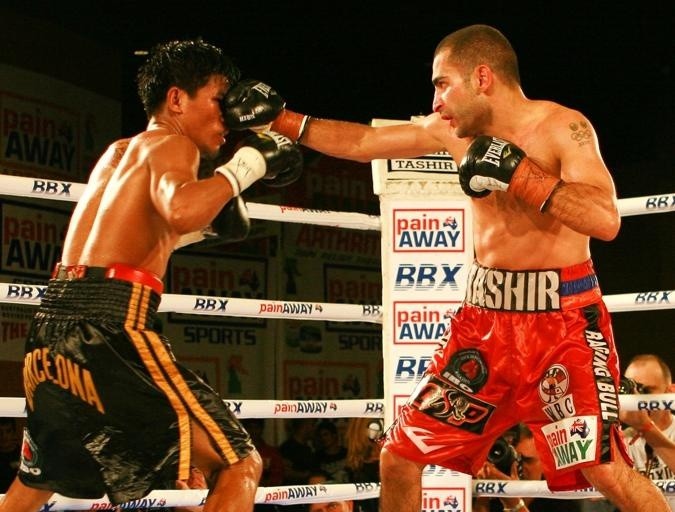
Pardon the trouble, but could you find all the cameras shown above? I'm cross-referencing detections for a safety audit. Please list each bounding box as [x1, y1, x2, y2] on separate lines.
[486, 436, 522, 476]
[618, 378, 650, 395]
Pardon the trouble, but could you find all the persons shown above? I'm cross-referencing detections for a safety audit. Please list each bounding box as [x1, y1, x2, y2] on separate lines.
[0, 38, 308, 512]
[0, 417, 23, 493]
[175, 350, 674, 512]
[221, 24, 673, 512]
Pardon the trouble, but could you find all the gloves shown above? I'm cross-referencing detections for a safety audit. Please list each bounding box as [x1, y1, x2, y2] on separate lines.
[219, 78, 312, 145]
[458, 136, 565, 215]
[171, 196, 251, 254]
[214, 131, 304, 198]
